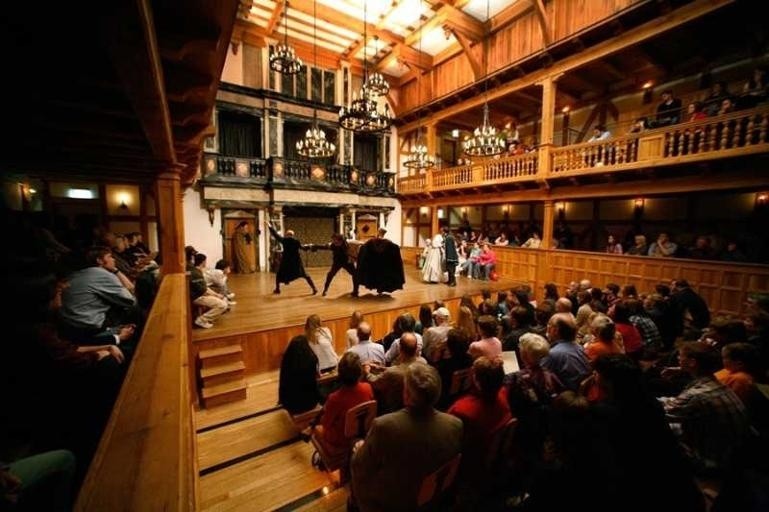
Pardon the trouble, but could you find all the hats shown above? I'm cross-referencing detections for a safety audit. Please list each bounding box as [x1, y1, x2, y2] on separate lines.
[431, 306, 450, 318]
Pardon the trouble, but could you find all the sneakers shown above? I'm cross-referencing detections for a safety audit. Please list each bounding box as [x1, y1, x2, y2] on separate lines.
[350, 290, 360, 296]
[311, 287, 319, 296]
[321, 289, 328, 297]
[221, 293, 237, 306]
[444, 281, 457, 287]
[272, 288, 281, 295]
[193, 316, 213, 329]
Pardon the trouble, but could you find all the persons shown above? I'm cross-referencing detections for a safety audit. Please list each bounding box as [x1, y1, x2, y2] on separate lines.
[305, 232, 366, 296]
[263, 220, 317, 295]
[233, 222, 253, 273]
[422, 228, 509, 287]
[694, 236, 741, 260]
[279, 278, 768, 511]
[350, 227, 405, 300]
[606, 231, 678, 258]
[500, 68, 768, 156]
[1, 214, 236, 512]
[513, 227, 560, 251]
